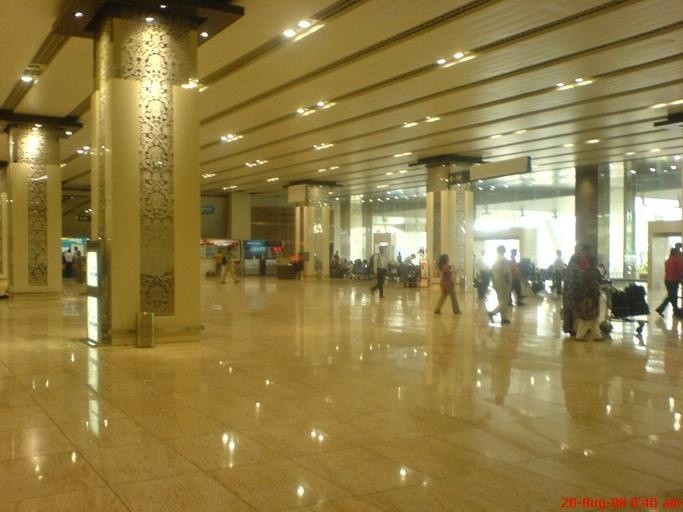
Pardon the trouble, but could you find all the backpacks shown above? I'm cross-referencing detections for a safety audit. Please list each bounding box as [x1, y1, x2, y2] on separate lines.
[624, 281, 649, 316]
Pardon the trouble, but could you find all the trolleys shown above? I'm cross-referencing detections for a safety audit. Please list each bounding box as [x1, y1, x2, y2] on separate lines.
[598, 275, 649, 335]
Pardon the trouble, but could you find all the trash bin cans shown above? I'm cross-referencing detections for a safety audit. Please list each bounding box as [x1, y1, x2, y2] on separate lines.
[135, 311, 154, 348]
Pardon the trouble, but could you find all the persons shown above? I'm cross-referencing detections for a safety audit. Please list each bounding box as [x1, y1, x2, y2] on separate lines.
[654, 248, 683, 317]
[63, 245, 86, 284]
[220, 248, 240, 284]
[329, 244, 611, 343]
[215, 250, 223, 275]
[674, 242, 683, 256]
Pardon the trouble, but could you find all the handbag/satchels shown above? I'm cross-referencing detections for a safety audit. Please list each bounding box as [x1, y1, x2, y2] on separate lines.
[611, 291, 627, 317]
